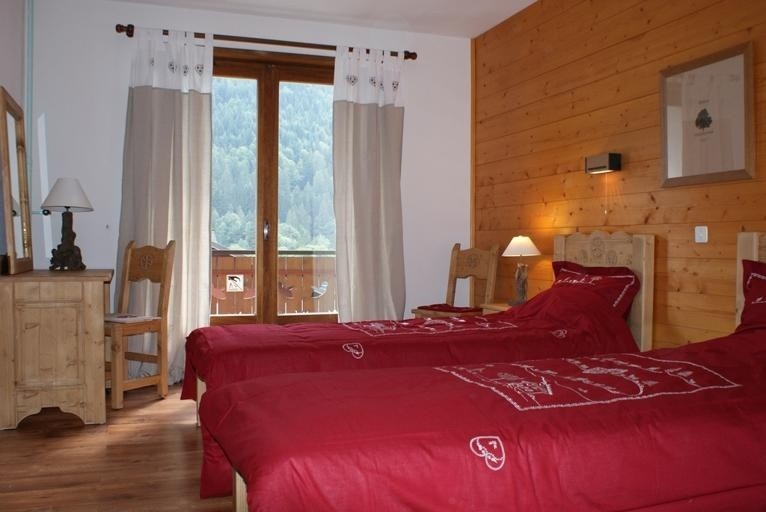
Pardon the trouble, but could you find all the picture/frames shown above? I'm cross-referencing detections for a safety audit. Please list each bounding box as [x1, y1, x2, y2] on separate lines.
[659, 39, 755, 188]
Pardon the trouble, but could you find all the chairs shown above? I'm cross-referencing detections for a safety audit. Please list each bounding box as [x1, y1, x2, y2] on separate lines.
[411, 241, 499, 319]
[104, 238, 176, 410]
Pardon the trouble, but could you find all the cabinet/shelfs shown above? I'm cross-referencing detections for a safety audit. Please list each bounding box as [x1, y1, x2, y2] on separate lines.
[0, 269, 115, 432]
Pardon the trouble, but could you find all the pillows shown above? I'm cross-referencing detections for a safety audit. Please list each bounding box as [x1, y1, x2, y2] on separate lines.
[550, 259, 642, 319]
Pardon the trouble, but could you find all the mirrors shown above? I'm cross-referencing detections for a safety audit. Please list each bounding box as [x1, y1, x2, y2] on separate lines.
[0, 84, 35, 276]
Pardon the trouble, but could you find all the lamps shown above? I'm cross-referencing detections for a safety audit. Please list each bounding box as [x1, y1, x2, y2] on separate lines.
[40, 177, 95, 271]
[584, 153, 622, 174]
[501, 234, 542, 306]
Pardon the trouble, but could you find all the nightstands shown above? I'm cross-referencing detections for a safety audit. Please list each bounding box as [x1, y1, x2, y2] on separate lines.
[477, 302, 521, 316]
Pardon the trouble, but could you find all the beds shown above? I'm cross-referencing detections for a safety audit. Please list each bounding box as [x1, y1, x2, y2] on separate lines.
[198, 230, 766, 512]
[182, 227, 656, 500]
